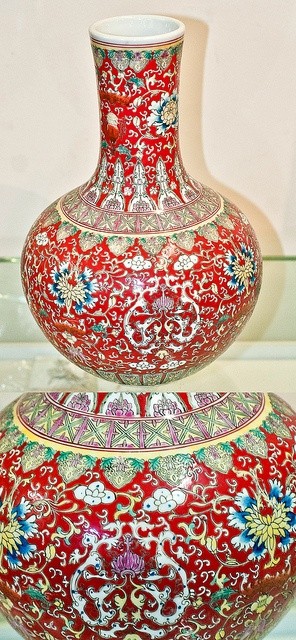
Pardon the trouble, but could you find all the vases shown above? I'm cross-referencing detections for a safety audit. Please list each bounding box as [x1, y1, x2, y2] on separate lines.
[19, 14, 264, 389]
[0, 391, 296, 640]
